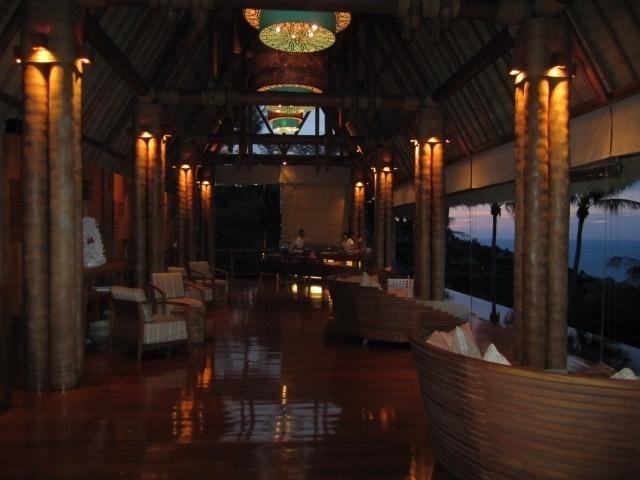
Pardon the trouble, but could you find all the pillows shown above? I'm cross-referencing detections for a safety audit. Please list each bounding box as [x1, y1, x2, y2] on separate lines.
[333, 272, 384, 291]
[609, 365, 638, 381]
[422, 320, 512, 369]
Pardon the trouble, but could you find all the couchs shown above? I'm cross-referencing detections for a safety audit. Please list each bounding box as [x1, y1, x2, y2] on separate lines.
[402, 315, 640, 480]
[323, 272, 466, 348]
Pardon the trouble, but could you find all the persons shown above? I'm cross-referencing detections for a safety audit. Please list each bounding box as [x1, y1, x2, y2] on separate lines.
[294, 230, 306, 254]
[342, 232, 355, 251]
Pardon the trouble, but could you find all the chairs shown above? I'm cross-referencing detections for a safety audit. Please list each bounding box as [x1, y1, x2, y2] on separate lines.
[104, 260, 230, 374]
[256, 245, 371, 311]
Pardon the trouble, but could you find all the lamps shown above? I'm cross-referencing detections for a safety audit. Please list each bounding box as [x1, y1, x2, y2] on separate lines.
[241, 5, 352, 135]
